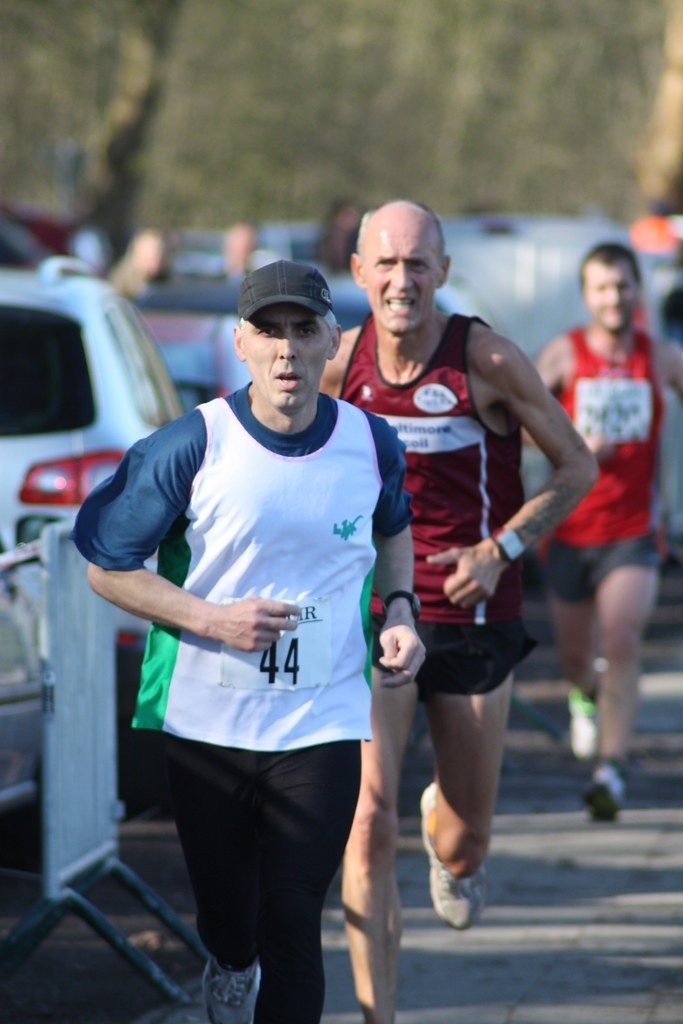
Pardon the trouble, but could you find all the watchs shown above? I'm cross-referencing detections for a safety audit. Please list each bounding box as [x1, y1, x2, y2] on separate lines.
[382, 590, 421, 620]
[491, 526, 525, 568]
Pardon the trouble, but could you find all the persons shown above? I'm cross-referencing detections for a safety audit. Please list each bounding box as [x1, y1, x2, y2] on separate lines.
[521, 243, 683, 818]
[71, 260, 426, 1024]
[211, 221, 258, 283]
[317, 199, 362, 270]
[317, 202, 598, 1023]
[108, 231, 178, 304]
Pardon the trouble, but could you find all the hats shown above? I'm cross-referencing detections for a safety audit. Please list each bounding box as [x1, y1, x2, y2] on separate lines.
[238, 260, 332, 320]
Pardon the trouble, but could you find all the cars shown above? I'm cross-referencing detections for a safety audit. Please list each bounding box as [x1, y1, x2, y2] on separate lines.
[0, 199, 623, 443]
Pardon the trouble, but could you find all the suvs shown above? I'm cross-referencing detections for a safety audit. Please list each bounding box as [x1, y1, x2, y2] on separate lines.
[0, 253, 188, 708]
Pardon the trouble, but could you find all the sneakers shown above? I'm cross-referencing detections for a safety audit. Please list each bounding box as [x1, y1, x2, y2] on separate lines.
[203, 955, 259, 1024]
[420, 781, 487, 929]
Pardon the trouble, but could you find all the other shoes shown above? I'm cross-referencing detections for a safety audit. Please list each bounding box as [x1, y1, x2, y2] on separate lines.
[568, 690, 597, 758]
[586, 780, 618, 819]
